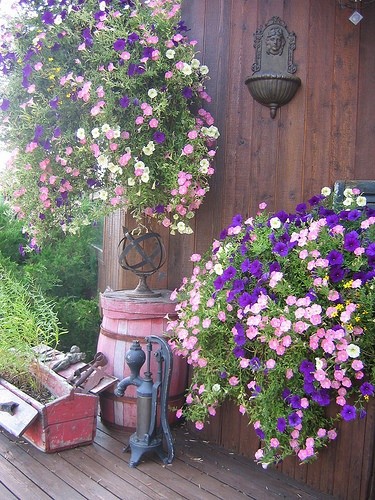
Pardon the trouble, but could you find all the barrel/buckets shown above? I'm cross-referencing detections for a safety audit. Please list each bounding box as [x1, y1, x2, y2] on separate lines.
[95, 290, 191, 432]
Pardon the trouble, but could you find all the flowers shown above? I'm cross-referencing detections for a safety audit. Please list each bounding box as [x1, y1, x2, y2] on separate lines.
[1, 1, 222, 256]
[161, 187, 375, 472]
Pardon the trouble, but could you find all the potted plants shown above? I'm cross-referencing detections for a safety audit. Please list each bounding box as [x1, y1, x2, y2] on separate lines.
[1, 260, 118, 454]
